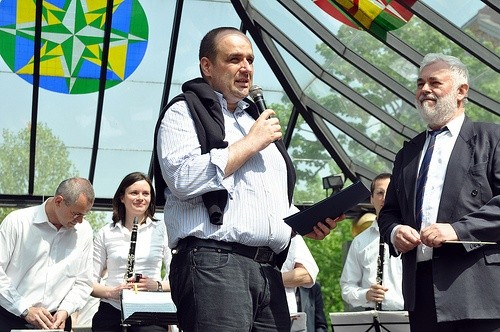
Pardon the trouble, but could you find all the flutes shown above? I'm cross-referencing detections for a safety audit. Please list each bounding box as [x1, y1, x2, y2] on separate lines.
[121, 216, 139, 327]
[375, 239, 385, 311]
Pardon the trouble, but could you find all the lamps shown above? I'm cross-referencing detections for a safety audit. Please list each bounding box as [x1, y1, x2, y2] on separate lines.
[322, 172, 345, 198]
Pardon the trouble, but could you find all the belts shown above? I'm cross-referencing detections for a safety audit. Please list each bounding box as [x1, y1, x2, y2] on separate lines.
[176, 235, 279, 266]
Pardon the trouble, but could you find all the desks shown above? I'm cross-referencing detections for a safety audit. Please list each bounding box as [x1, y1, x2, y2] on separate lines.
[120, 289, 177, 332]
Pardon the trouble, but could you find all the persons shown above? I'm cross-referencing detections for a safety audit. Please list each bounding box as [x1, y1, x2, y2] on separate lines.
[377, 52, 500, 332]
[0, 177, 95, 332]
[154, 26, 345, 332]
[283, 173, 412, 332]
[91, 172, 173, 332]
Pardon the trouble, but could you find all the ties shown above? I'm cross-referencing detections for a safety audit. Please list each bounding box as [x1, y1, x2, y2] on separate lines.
[414, 126, 449, 232]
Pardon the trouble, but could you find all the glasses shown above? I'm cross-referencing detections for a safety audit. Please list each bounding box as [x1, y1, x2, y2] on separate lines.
[61, 194, 91, 218]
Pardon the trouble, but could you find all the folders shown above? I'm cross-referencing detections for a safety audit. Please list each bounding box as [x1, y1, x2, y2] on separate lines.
[283, 181, 373, 236]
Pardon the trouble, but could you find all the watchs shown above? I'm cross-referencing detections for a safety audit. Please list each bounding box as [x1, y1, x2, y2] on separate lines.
[156, 281, 162, 292]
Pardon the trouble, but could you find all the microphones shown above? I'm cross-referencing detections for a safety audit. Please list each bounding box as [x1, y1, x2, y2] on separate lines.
[249, 84, 271, 120]
